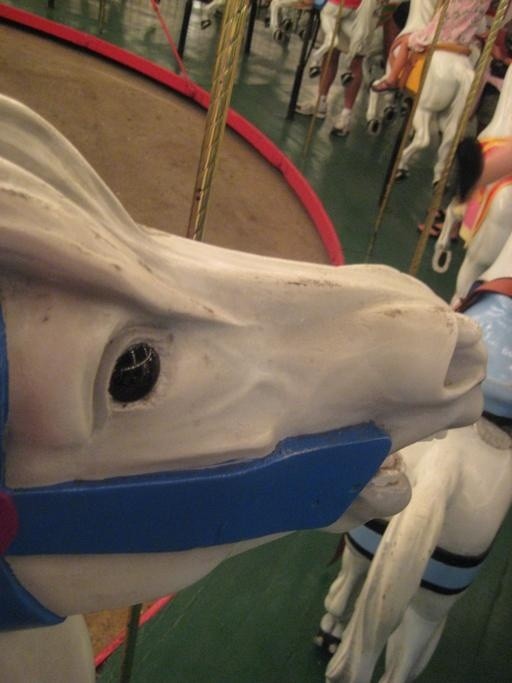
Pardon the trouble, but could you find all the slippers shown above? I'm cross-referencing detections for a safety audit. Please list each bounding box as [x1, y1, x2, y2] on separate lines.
[373, 78, 399, 92]
[418, 222, 459, 243]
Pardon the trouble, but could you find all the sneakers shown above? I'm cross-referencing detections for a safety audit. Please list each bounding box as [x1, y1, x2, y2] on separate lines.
[334, 115, 356, 136]
[295, 100, 327, 119]
